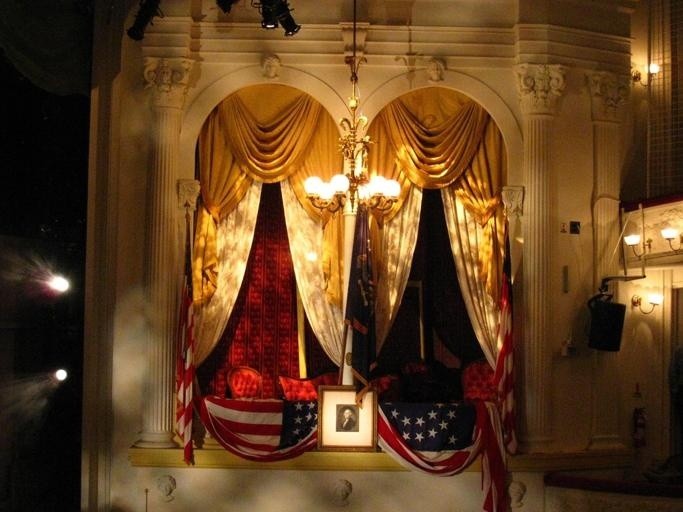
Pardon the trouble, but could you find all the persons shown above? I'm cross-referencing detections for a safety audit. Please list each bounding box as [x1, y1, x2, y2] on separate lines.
[341, 409, 356, 430]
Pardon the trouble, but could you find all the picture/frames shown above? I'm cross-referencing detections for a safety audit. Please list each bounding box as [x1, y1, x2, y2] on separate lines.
[317, 385, 379, 453]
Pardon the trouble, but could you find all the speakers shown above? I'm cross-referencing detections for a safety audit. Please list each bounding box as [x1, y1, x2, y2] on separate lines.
[588, 299, 626, 352]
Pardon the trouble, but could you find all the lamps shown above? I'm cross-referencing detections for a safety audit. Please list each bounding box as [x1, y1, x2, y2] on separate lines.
[632, 293, 664, 316]
[632, 64, 660, 87]
[300, 0, 403, 215]
[623, 228, 683, 257]
